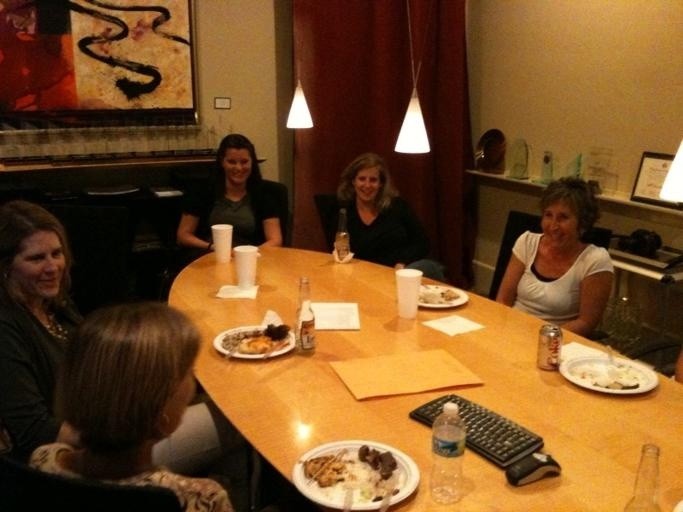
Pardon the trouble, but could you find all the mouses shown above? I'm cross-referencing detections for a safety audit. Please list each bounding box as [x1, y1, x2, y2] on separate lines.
[506, 452, 562, 486]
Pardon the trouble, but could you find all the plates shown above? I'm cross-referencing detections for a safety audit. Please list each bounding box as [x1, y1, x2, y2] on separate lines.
[509, 138, 528, 179]
[559, 355, 660, 395]
[419, 284, 470, 310]
[292, 440, 421, 512]
[476, 128, 507, 167]
[212, 325, 295, 360]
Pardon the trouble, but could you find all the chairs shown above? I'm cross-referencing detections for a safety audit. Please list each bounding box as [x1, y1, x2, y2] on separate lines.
[0, 461, 179, 512]
[308, 190, 409, 265]
[483, 209, 615, 342]
[234, 178, 292, 251]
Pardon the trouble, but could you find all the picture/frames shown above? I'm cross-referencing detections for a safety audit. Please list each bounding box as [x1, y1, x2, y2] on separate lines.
[0, 2, 198, 124]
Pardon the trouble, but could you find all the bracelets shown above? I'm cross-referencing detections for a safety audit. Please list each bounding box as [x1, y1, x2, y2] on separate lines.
[208, 241, 214, 251]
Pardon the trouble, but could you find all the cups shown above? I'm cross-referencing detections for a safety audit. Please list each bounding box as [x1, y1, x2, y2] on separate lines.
[233, 245, 258, 292]
[212, 223, 233, 266]
[396, 268, 422, 320]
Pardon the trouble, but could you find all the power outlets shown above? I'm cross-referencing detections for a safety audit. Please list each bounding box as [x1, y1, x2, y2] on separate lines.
[215, 98, 231, 109]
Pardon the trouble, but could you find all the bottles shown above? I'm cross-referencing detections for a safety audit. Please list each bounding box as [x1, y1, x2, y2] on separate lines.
[294, 276, 316, 353]
[623, 444, 662, 512]
[336, 209, 350, 261]
[537, 324, 563, 371]
[429, 402, 467, 506]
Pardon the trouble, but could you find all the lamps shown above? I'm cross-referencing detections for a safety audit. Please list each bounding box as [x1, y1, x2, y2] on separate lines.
[659, 137, 683, 205]
[285, 86, 313, 129]
[394, 90, 433, 155]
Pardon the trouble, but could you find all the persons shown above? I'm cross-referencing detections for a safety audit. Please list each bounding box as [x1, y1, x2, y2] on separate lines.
[496, 176, 614, 336]
[338, 153, 407, 269]
[28, 304, 234, 512]
[0, 201, 244, 473]
[176, 134, 284, 248]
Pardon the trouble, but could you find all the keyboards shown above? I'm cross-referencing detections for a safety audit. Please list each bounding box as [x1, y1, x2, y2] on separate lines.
[408, 394, 544, 468]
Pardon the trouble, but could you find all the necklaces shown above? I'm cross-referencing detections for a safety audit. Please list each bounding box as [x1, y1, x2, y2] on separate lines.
[35, 310, 67, 339]
